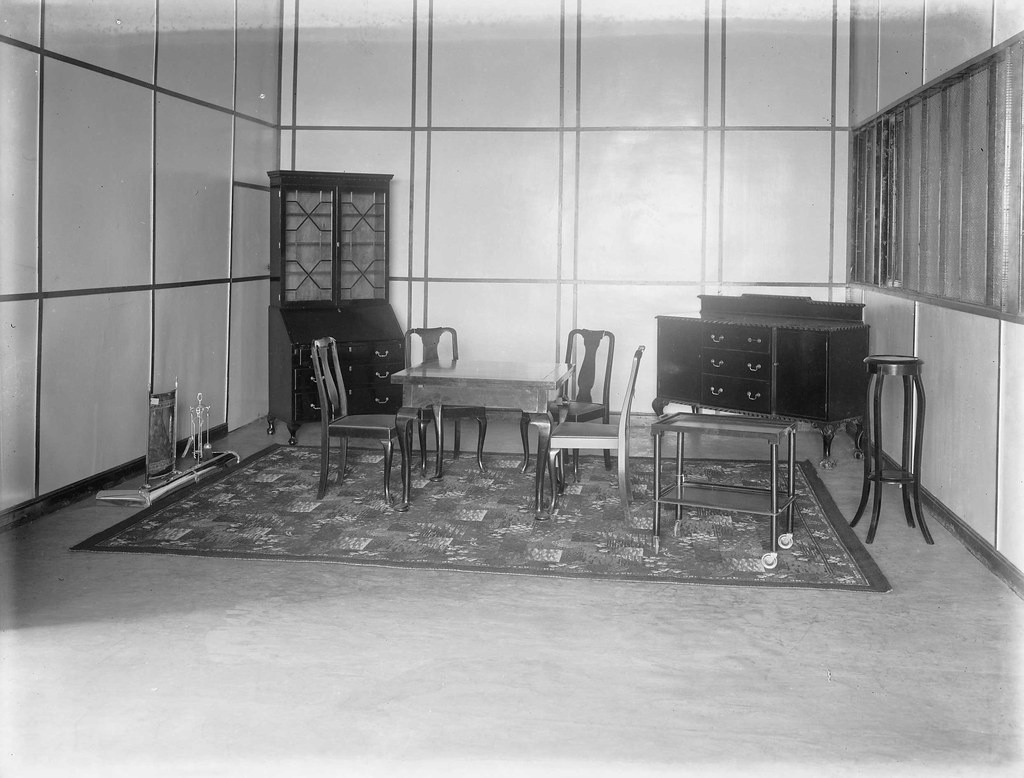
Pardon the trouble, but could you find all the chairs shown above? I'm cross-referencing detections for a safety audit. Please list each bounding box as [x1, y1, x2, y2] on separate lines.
[548, 345, 647, 517]
[312, 336, 412, 506]
[521, 330, 616, 474]
[405, 326, 488, 477]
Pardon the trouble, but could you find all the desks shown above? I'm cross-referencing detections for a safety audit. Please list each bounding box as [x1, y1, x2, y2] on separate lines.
[386, 357, 578, 520]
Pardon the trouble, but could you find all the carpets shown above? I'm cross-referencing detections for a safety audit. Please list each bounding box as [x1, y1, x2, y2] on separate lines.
[73, 444, 895, 596]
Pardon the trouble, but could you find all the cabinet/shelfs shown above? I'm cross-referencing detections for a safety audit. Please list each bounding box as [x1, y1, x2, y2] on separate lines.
[849, 355, 934, 545]
[646, 409, 802, 566]
[266, 170, 408, 448]
[658, 292, 869, 467]
